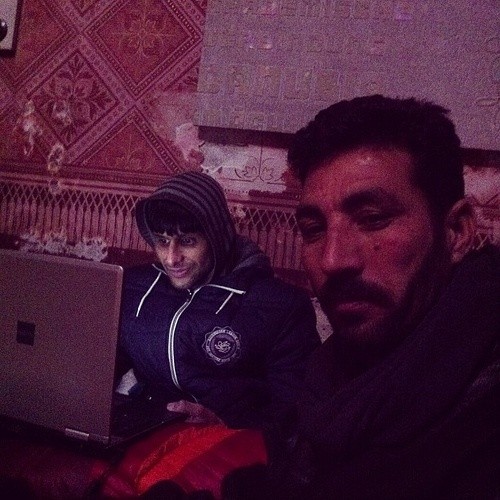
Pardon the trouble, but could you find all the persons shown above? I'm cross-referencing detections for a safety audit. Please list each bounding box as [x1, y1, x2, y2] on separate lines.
[112, 171, 316, 447]
[280, 94, 500, 500]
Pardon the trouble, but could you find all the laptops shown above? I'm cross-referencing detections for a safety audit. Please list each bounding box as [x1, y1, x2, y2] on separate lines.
[0, 250, 188, 449]
